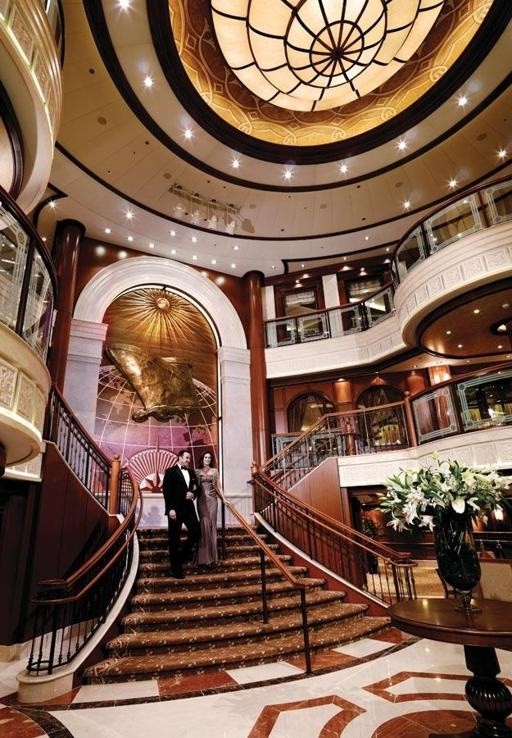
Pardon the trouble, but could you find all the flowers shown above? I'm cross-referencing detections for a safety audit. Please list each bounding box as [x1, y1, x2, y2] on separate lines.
[369, 441, 512, 533]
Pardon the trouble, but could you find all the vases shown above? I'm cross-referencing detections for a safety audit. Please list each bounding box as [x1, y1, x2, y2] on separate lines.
[434, 509, 483, 613]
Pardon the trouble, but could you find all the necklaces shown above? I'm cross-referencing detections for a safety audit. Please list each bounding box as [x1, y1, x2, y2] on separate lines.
[202, 468, 209, 475]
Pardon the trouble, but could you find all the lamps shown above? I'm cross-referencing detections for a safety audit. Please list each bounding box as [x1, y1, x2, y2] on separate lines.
[168, 181, 243, 237]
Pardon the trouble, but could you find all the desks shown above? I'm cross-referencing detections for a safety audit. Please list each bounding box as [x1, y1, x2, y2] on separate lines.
[384, 597, 511, 737]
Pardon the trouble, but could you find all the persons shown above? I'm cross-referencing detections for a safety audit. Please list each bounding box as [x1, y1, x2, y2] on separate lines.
[193, 449, 229, 566]
[162, 449, 202, 579]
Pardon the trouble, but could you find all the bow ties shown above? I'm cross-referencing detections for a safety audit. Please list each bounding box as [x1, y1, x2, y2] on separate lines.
[181, 466, 188, 470]
